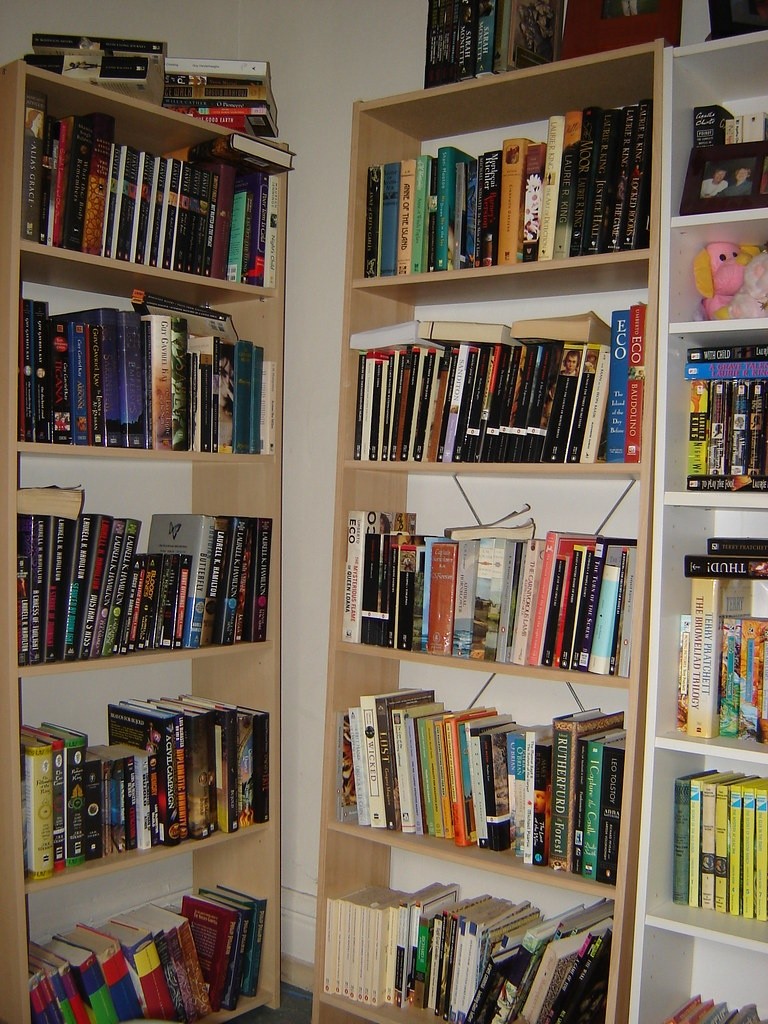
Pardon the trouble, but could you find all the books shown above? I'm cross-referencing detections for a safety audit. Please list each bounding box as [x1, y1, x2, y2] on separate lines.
[15, 33, 295, 1024]
[324, 0, 768, 1024]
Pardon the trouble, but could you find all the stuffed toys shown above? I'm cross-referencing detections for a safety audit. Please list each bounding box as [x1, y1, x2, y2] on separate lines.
[691, 242, 759, 323]
[729, 252, 768, 319]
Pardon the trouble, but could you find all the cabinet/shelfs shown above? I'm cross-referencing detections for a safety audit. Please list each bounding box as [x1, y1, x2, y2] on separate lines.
[0, 61, 290, 1024]
[311, 30, 768, 1024]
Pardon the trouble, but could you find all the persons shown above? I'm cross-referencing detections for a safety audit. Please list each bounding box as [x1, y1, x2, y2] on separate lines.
[719, 165, 752, 198]
[700, 166, 729, 199]
[560, 350, 580, 377]
[534, 791, 545, 813]
[583, 352, 597, 372]
